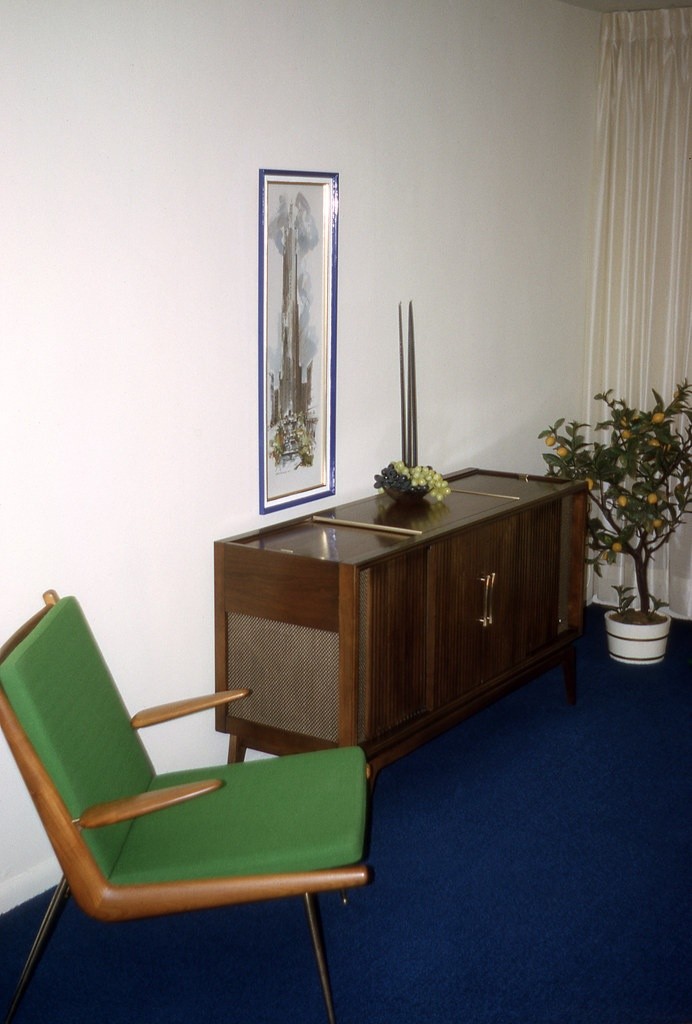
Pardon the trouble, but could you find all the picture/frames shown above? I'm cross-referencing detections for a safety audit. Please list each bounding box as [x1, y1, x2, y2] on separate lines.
[258, 168, 339, 515]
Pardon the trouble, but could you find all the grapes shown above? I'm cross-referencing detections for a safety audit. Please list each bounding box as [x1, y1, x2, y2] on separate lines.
[373, 460, 451, 502]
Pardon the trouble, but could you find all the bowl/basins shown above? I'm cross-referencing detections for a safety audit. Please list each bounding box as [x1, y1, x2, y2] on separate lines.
[382, 483, 435, 504]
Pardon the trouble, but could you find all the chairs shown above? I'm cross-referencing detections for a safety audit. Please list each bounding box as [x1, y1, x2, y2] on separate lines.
[0, 590, 374, 1024]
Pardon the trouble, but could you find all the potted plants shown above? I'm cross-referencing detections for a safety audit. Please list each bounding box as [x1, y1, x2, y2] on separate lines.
[538, 378, 692, 665]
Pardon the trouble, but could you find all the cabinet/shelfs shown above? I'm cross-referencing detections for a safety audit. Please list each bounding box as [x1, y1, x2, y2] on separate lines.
[214, 467, 589, 802]
